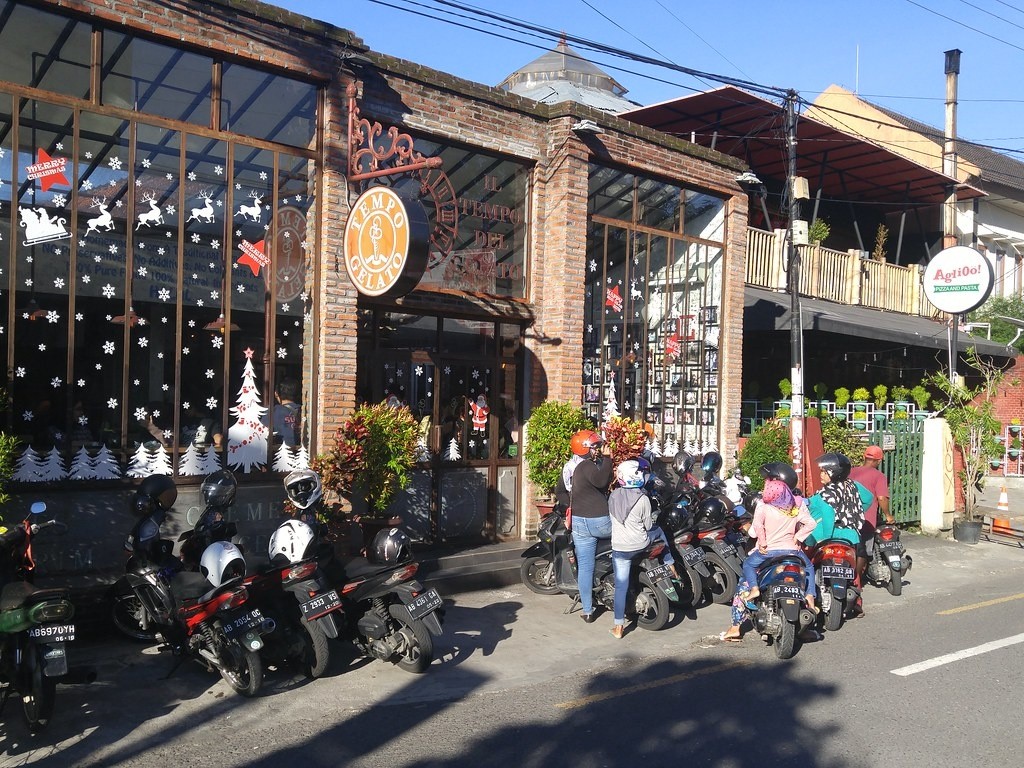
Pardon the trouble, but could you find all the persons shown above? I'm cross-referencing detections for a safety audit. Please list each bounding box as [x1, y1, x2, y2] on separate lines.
[139, 391, 223, 446]
[743, 462, 819, 614]
[261, 376, 301, 446]
[554, 430, 612, 622]
[608, 457, 683, 640]
[721, 488, 818, 642]
[801, 453, 875, 617]
[48, 393, 92, 450]
[849, 445, 895, 557]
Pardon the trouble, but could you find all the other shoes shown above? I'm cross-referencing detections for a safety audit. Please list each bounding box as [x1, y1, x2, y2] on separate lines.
[579, 615, 592, 623]
[609, 625, 623, 638]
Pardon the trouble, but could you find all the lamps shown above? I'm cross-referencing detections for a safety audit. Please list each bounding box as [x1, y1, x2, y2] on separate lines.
[735, 172, 762, 184]
[111, 80, 150, 326]
[16, 55, 51, 320]
[571, 120, 604, 135]
[203, 102, 240, 333]
[340, 52, 375, 66]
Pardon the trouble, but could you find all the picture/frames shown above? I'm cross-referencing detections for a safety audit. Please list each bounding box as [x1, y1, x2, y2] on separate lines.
[582, 306, 719, 426]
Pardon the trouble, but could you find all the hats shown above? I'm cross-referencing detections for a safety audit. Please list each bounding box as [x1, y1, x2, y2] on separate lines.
[863, 445, 884, 459]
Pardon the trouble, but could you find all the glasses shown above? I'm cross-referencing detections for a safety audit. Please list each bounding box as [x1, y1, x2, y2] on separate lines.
[590, 445, 599, 451]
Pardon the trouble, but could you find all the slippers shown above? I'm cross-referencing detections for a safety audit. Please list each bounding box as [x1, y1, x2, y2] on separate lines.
[720, 632, 742, 642]
[740, 597, 758, 610]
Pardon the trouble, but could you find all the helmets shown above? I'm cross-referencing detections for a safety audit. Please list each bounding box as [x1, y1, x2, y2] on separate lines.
[658, 503, 690, 532]
[137, 474, 177, 512]
[758, 462, 798, 492]
[701, 452, 722, 473]
[199, 541, 246, 588]
[571, 430, 603, 459]
[284, 469, 322, 509]
[671, 450, 696, 478]
[372, 528, 411, 566]
[201, 471, 237, 507]
[617, 460, 650, 488]
[693, 498, 727, 526]
[268, 519, 315, 566]
[814, 452, 851, 484]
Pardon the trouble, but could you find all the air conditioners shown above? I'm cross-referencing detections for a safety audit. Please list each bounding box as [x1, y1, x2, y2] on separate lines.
[649, 262, 709, 284]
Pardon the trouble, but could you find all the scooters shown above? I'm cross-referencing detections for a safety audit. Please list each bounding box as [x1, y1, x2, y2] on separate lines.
[0, 502, 75, 734]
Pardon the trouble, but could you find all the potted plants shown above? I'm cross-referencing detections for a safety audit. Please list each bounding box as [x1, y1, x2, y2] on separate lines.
[523, 398, 594, 520]
[777, 333, 1021, 544]
[345, 400, 426, 545]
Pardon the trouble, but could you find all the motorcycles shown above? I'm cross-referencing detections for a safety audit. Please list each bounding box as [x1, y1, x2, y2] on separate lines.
[646, 452, 749, 610]
[518, 497, 679, 631]
[102, 473, 278, 698]
[174, 477, 343, 678]
[732, 505, 818, 660]
[861, 521, 914, 596]
[291, 495, 449, 675]
[791, 486, 859, 633]
